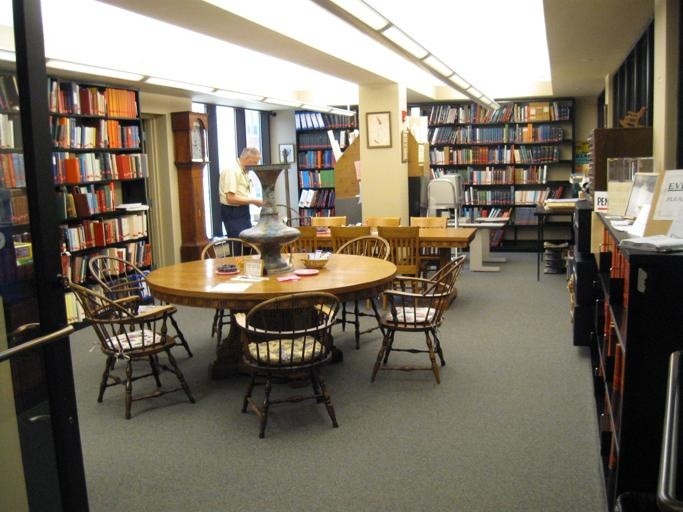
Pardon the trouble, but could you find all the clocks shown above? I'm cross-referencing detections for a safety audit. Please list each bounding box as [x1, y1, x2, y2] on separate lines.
[169, 109, 215, 263]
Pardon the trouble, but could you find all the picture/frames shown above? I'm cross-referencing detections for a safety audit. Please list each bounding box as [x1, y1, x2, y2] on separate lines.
[399, 129, 407, 164]
[365, 109, 394, 151]
[277, 143, 295, 164]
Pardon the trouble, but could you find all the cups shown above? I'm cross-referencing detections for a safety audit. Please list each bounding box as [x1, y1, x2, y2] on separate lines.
[606, 156, 654, 217]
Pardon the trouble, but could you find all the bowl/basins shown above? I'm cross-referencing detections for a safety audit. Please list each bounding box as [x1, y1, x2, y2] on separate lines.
[303, 258, 328, 268]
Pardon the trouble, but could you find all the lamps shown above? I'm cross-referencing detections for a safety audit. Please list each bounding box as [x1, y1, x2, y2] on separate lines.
[317, 0, 504, 111]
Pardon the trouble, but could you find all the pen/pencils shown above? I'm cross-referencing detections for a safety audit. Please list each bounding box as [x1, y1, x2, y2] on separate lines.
[240, 276, 260, 280]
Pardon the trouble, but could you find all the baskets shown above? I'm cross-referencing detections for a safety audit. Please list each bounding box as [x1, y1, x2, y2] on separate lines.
[301, 259, 328, 269]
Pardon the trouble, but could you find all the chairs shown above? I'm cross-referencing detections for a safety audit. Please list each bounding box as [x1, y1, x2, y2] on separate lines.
[147, 235, 396, 374]
[233, 292, 341, 439]
[370, 255, 468, 385]
[298, 216, 477, 310]
[70, 255, 195, 419]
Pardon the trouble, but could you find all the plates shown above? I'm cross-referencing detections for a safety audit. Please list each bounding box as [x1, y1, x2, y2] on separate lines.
[292, 268, 320, 276]
[216, 269, 240, 274]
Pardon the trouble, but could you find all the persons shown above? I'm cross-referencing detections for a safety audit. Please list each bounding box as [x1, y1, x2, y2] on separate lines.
[218, 147, 267, 256]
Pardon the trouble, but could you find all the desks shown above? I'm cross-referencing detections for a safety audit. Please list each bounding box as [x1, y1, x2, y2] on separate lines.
[447, 217, 510, 274]
[531, 206, 577, 280]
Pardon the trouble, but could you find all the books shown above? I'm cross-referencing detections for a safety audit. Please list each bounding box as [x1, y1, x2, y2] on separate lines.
[296, 108, 355, 231]
[421, 97, 577, 249]
[0, 69, 154, 325]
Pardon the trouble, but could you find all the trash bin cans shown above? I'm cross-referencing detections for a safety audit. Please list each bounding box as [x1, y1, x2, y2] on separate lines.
[543, 241, 568, 274]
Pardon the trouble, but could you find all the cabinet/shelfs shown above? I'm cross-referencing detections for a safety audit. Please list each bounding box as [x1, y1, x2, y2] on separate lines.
[585, 203, 680, 512]
[585, 123, 652, 193]
[290, 94, 577, 255]
[0, 62, 155, 331]
[568, 195, 598, 350]
